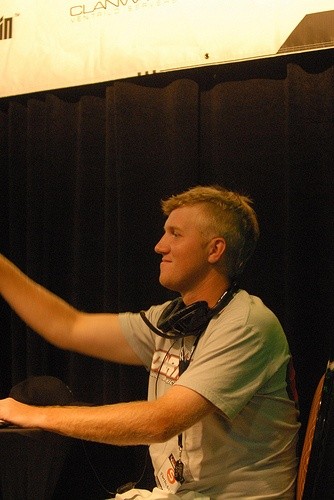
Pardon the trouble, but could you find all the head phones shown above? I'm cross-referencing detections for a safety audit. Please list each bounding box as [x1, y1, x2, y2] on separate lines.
[157, 278, 241, 335]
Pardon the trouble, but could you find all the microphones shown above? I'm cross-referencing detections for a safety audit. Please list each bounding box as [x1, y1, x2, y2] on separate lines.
[140, 310, 180, 339]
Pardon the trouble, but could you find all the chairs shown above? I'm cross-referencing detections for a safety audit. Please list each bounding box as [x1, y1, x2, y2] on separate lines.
[295, 361, 334, 500]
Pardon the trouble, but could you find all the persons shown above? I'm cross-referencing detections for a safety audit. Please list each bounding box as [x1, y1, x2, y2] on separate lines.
[0, 186, 300, 500]
